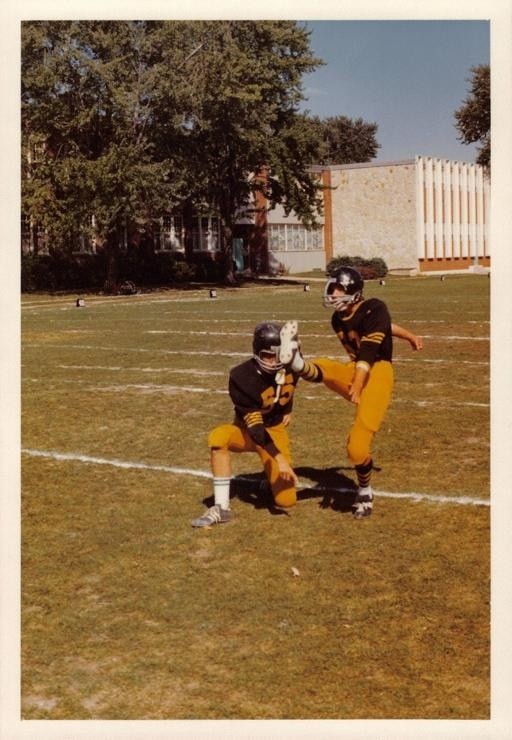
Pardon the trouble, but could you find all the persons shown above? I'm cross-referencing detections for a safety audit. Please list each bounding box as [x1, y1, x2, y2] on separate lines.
[279, 266, 422, 520]
[190, 324, 299, 527]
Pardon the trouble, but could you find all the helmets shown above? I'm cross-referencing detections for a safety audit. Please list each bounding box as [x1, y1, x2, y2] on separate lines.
[324, 267, 363, 302]
[252, 323, 282, 361]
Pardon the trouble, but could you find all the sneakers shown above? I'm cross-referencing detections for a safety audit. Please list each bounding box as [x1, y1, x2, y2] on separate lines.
[351, 492, 374, 519]
[189, 504, 232, 526]
[259, 470, 272, 498]
[279, 320, 299, 365]
